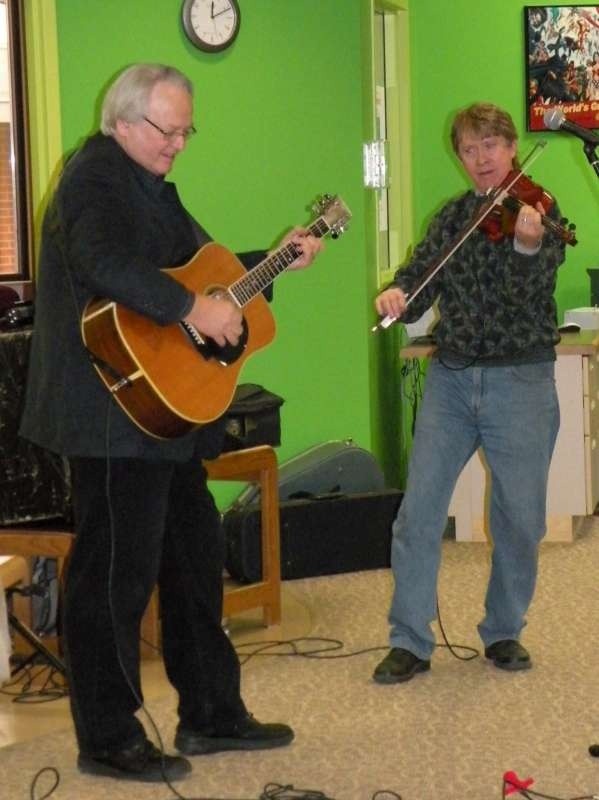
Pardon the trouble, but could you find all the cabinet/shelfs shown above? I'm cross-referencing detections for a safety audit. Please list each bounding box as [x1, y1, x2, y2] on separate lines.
[399, 329, 599, 541]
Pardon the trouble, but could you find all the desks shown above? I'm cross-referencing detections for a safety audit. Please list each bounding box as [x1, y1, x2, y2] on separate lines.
[141, 445, 281, 657]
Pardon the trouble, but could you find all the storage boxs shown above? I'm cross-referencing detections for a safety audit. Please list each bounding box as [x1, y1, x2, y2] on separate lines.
[565, 307, 599, 329]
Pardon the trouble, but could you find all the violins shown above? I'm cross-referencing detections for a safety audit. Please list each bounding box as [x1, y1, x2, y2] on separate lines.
[371, 137, 577, 340]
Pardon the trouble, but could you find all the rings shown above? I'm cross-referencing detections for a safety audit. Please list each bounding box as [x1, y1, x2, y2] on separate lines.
[522, 216, 527, 224]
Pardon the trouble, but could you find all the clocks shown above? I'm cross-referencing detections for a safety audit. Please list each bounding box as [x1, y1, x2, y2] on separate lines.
[182, 0, 241, 53]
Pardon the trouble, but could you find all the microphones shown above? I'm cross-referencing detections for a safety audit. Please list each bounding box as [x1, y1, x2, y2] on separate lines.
[544, 110, 599, 145]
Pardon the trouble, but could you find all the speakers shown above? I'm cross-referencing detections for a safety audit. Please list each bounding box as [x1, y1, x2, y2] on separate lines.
[0, 327, 75, 530]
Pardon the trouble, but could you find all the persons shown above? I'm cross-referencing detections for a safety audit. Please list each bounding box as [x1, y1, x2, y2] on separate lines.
[371, 104, 567, 683]
[18, 63, 326, 783]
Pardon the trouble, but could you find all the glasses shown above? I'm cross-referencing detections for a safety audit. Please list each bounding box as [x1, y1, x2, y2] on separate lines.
[144, 118, 196, 143]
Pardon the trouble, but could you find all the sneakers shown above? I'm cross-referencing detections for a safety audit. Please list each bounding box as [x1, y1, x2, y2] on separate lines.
[484, 640, 531, 669]
[173, 711, 295, 755]
[76, 738, 193, 782]
[373, 648, 431, 683]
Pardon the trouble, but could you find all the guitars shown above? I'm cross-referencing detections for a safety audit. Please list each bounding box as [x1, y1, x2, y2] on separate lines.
[81, 193, 352, 444]
